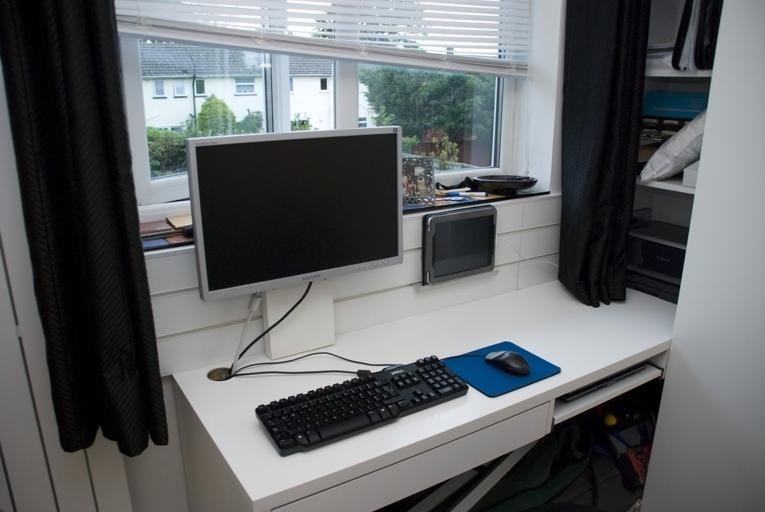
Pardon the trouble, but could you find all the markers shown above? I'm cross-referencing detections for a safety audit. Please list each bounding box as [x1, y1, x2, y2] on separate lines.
[447, 191, 487, 197]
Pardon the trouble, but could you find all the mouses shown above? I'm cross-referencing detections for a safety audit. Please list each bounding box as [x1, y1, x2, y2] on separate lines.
[484, 351, 529, 377]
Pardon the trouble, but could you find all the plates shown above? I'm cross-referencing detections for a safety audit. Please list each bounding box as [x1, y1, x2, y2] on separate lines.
[473, 174, 539, 195]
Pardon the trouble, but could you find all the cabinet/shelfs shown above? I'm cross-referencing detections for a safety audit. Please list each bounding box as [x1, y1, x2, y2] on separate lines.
[627, 1, 765, 509]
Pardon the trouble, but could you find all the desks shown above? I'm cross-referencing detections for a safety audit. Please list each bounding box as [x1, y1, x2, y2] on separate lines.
[172, 279, 677, 512]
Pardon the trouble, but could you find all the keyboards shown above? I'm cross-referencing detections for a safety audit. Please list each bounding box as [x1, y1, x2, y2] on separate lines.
[255, 355, 469, 457]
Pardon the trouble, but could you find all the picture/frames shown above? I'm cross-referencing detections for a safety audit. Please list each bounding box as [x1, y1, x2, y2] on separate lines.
[404, 158, 436, 210]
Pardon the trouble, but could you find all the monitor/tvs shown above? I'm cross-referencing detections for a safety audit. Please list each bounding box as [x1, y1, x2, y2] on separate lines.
[185, 125, 404, 302]
[423, 205, 497, 285]
